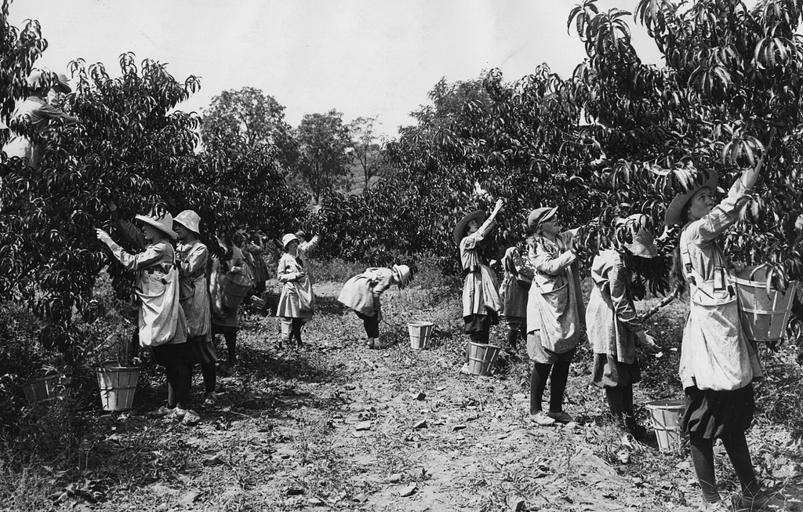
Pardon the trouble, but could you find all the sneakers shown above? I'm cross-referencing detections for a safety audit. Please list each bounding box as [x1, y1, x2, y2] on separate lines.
[548, 411, 574, 423]
[740, 492, 795, 510]
[700, 497, 732, 512]
[198, 393, 221, 410]
[529, 411, 556, 424]
[365, 341, 391, 350]
[147, 403, 191, 419]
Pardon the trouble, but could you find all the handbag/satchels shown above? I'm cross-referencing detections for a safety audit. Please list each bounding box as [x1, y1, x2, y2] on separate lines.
[534, 235, 580, 354]
[179, 244, 211, 336]
[136, 240, 181, 348]
[680, 220, 753, 391]
[293, 275, 315, 312]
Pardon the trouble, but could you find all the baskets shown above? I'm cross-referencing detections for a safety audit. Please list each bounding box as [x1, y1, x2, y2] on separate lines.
[221, 271, 253, 309]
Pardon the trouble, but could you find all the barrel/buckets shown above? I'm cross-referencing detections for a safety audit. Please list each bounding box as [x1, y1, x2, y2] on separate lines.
[644, 397, 693, 454]
[282, 320, 294, 341]
[96, 364, 142, 412]
[407, 320, 434, 349]
[467, 342, 501, 375]
[731, 263, 798, 343]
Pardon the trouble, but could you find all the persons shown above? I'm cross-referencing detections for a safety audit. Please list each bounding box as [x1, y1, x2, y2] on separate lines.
[584, 213, 661, 443]
[525, 207, 585, 427]
[337, 263, 411, 351]
[500, 248, 531, 351]
[453, 201, 503, 345]
[95, 205, 269, 425]
[664, 131, 776, 511]
[277, 233, 319, 348]
[296, 230, 307, 248]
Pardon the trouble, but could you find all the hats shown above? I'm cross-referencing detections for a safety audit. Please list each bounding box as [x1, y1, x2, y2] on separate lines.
[172, 209, 202, 236]
[663, 174, 720, 227]
[610, 213, 659, 259]
[453, 208, 485, 248]
[132, 207, 178, 239]
[392, 263, 411, 288]
[282, 232, 301, 249]
[527, 204, 560, 234]
